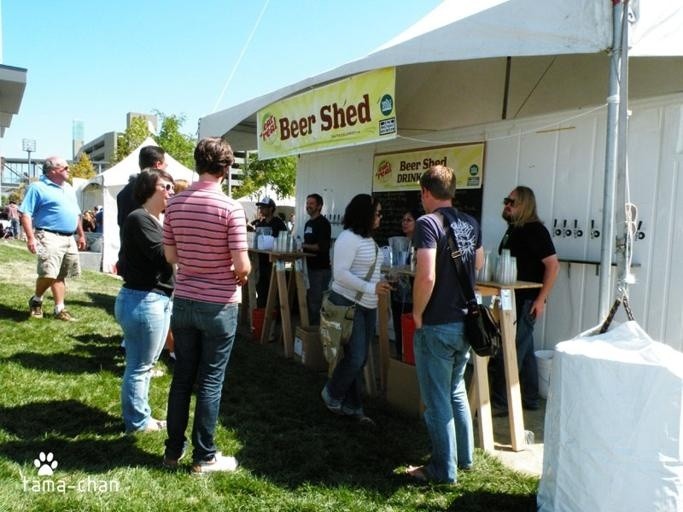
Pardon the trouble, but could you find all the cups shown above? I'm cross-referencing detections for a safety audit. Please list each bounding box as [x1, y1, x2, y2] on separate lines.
[247, 226, 296, 253]
[396, 251, 409, 269]
[477, 248, 518, 285]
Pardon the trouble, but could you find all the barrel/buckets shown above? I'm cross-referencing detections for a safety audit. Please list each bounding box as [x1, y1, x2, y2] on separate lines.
[534, 349, 554, 398]
[390, 236, 410, 265]
[253, 308, 277, 343]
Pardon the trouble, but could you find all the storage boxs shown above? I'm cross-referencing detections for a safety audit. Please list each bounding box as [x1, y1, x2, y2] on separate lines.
[294, 324, 331, 373]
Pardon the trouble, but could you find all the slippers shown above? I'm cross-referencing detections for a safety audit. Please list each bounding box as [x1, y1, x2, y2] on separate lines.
[406, 465, 437, 481]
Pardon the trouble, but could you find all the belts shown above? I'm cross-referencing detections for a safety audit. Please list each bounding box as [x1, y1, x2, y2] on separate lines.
[36, 227, 74, 236]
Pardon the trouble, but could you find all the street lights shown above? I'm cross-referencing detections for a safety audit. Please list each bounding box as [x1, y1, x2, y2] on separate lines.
[21, 138, 37, 179]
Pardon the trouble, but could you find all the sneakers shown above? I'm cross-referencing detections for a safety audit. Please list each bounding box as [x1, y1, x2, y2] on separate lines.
[149, 369, 164, 378]
[477, 401, 509, 419]
[524, 399, 541, 411]
[53, 309, 78, 323]
[342, 404, 364, 417]
[163, 448, 186, 467]
[191, 454, 238, 476]
[322, 386, 342, 413]
[28, 296, 44, 320]
[135, 417, 168, 433]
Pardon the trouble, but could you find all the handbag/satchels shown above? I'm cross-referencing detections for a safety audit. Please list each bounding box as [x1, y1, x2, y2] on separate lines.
[319, 289, 356, 379]
[465, 303, 502, 358]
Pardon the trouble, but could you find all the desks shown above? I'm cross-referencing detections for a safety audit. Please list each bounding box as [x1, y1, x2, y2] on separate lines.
[232, 250, 317, 356]
[364, 265, 547, 455]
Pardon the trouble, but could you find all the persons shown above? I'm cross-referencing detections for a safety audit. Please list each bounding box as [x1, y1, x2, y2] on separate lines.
[17, 157, 86, 322]
[117, 145, 176, 378]
[162, 138, 252, 478]
[114, 167, 176, 433]
[0, 200, 103, 239]
[174, 179, 188, 194]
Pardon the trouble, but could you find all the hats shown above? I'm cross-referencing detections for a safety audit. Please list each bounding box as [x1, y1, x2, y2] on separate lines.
[256, 196, 276, 208]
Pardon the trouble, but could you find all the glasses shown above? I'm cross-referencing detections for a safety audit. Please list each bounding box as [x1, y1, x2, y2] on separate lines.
[374, 210, 384, 218]
[59, 164, 70, 173]
[401, 218, 418, 225]
[155, 182, 175, 193]
[504, 197, 517, 209]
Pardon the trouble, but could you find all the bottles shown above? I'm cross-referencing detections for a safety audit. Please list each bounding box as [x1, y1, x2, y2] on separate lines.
[408, 246, 417, 273]
[35, 243, 48, 260]
